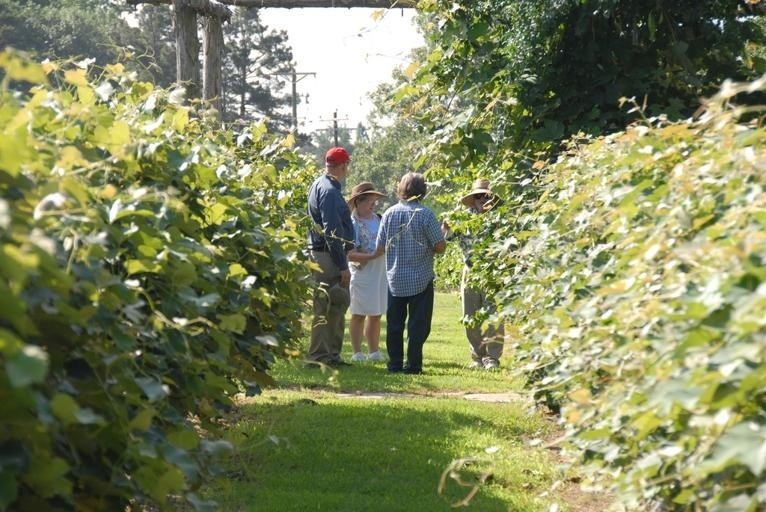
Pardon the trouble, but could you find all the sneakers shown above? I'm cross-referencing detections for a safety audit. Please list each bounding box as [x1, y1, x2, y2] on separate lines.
[305, 350, 502, 376]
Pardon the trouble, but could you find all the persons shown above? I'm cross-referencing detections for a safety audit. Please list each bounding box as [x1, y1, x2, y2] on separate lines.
[440, 177, 507, 371]
[375, 171, 449, 373]
[306, 147, 355, 367]
[343, 182, 389, 364]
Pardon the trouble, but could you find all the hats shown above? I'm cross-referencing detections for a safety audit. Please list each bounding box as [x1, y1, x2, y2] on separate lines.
[325, 146, 352, 167]
[346, 181, 386, 208]
[462, 178, 507, 208]
